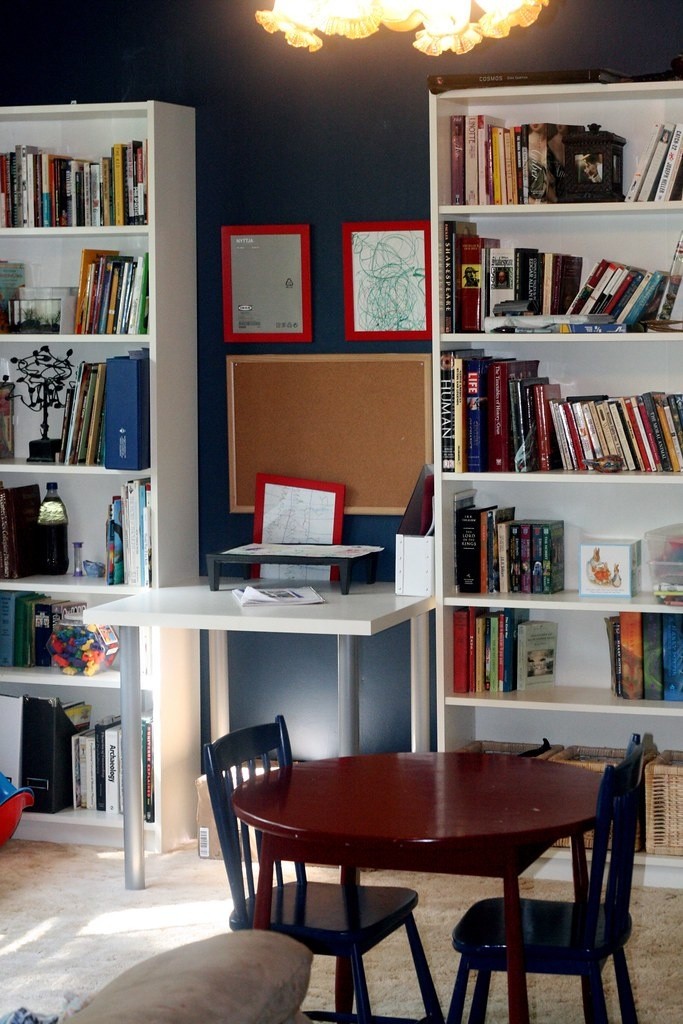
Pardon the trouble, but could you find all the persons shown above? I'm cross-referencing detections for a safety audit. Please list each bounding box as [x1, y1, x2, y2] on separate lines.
[548, 124, 573, 202]
[528, 123, 547, 203]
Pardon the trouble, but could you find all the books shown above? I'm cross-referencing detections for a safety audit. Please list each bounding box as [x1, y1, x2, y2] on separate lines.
[443, 221, 670, 334]
[453, 606, 558, 694]
[59, 362, 107, 465]
[74, 249, 149, 335]
[0, 382, 14, 458]
[624, 122, 683, 201]
[106, 478, 152, 588]
[453, 490, 565, 595]
[0, 590, 87, 669]
[59, 701, 154, 823]
[0, 260, 25, 334]
[0, 481, 43, 579]
[450, 114, 584, 206]
[0, 140, 149, 229]
[604, 612, 683, 702]
[441, 349, 683, 474]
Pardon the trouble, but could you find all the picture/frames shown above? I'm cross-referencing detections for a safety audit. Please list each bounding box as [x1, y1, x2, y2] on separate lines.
[341, 220, 431, 341]
[220, 223, 313, 343]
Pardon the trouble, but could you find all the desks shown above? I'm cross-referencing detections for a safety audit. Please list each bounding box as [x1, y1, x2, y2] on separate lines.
[83, 573, 435, 891]
[230, 752, 608, 1024]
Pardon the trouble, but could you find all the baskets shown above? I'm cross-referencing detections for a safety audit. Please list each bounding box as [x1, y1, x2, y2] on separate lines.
[460, 740, 683, 857]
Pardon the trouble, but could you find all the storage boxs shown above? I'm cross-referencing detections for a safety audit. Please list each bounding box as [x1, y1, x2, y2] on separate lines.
[195, 758, 339, 867]
[578, 538, 641, 598]
[644, 520, 683, 607]
[395, 534, 435, 598]
[562, 120, 626, 203]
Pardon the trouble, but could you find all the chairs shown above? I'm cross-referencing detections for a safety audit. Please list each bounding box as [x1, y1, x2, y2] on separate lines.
[447, 746, 643, 1024]
[203, 714, 446, 1024]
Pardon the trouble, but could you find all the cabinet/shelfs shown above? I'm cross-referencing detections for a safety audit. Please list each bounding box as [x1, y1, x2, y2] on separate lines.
[431, 77, 683, 890]
[0, 100, 198, 856]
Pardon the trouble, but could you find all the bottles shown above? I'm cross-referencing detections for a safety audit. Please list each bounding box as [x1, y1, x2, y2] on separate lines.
[35, 482, 70, 575]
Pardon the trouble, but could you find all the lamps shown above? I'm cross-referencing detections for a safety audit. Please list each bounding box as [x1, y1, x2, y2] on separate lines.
[255, 0, 551, 56]
